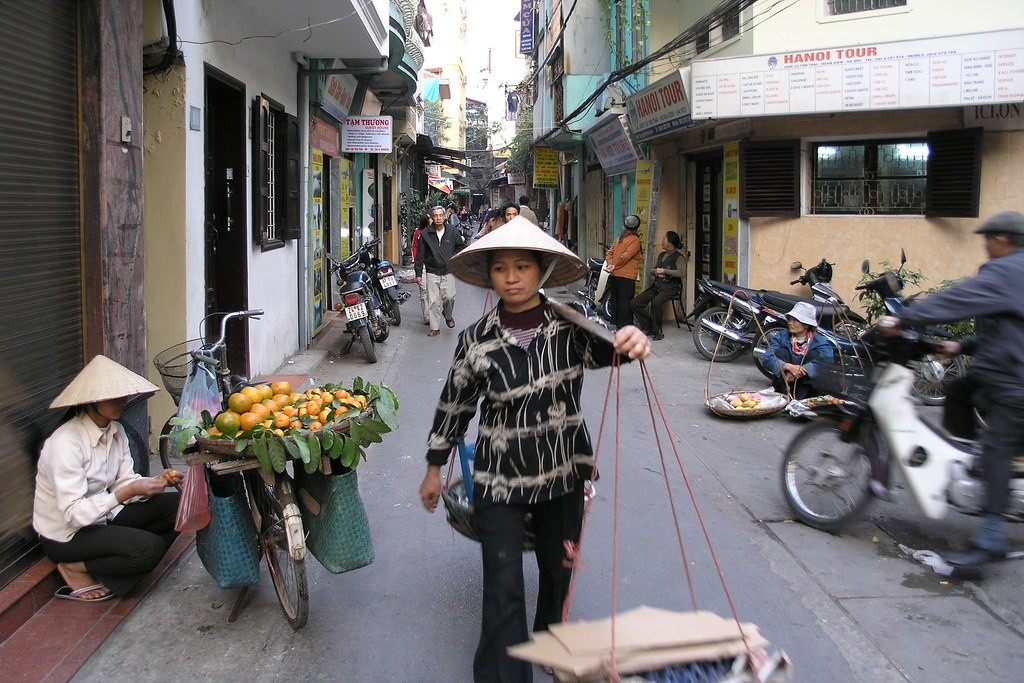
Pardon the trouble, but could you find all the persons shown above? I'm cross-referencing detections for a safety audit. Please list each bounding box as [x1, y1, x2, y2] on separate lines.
[603, 215, 641, 331]
[474, 195, 538, 239]
[411, 205, 468, 336]
[878, 211, 1024, 567]
[630, 231, 687, 341]
[762, 302, 834, 413]
[419, 215, 651, 683]
[32, 354, 186, 600]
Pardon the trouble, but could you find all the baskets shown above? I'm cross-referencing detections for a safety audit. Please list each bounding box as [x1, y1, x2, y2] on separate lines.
[194, 390, 374, 458]
[704, 391, 790, 420]
[444, 474, 535, 550]
[153, 337, 224, 407]
[793, 396, 855, 423]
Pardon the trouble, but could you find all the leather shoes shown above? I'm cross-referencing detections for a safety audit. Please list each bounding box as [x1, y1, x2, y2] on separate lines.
[426, 330, 440, 337]
[443, 311, 456, 328]
[949, 545, 1006, 565]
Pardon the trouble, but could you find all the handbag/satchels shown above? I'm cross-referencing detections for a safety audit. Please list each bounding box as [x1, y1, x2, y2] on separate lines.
[194, 470, 258, 587]
[301, 460, 376, 575]
[175, 464, 212, 532]
[174, 351, 221, 444]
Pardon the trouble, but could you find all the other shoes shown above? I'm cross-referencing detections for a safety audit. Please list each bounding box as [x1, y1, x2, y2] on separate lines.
[653, 333, 664, 341]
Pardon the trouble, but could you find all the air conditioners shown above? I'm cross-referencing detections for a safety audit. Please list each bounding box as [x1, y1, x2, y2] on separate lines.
[595, 73, 623, 116]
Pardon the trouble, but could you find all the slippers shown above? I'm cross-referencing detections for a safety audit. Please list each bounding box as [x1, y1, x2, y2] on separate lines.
[54, 580, 116, 601]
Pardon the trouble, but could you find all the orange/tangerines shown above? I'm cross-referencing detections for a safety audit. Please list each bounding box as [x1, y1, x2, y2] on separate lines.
[208, 380, 368, 438]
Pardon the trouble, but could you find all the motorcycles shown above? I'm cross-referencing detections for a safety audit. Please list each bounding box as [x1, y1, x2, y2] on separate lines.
[682, 256, 867, 364]
[577, 242, 613, 322]
[779, 319, 1024, 536]
[749, 247, 966, 407]
[326, 225, 413, 363]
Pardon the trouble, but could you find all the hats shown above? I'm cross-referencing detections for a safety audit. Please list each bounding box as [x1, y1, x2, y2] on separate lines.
[785, 301, 817, 328]
[624, 214, 640, 228]
[449, 215, 590, 289]
[48, 353, 161, 408]
[974, 210, 1024, 233]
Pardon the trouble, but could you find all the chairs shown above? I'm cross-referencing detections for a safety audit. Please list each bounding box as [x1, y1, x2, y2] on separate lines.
[658, 280, 692, 333]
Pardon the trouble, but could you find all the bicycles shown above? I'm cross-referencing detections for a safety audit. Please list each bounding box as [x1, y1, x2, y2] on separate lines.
[153, 309, 310, 631]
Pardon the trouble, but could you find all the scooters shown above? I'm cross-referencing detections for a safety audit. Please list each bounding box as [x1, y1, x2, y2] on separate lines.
[455, 221, 475, 246]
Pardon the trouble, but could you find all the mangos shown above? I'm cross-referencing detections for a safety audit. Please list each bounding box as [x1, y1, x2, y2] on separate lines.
[730, 394, 761, 409]
